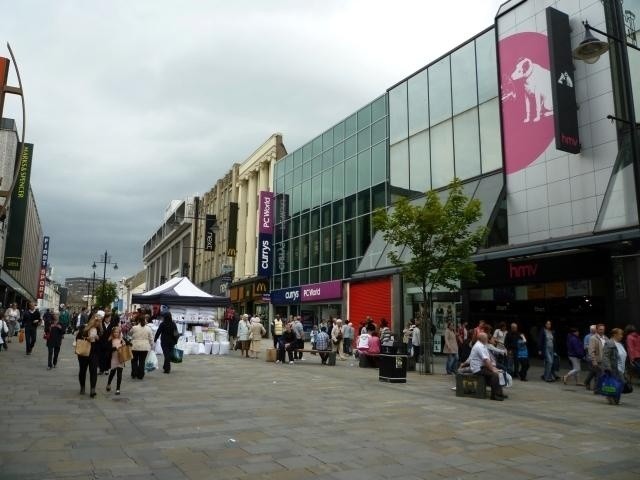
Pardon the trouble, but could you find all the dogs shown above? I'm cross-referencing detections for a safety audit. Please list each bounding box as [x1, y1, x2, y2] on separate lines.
[510, 56, 553, 123]
[19, 329, 24, 343]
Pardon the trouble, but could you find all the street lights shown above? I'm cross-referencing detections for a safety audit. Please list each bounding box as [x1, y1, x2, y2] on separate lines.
[83, 250, 118, 311]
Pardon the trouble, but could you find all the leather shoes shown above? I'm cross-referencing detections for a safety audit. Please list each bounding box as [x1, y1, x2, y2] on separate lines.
[491, 392, 508, 401]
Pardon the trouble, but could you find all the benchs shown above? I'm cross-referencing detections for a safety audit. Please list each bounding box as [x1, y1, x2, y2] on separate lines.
[456, 372, 487, 399]
[357, 351, 391, 369]
[273, 345, 339, 368]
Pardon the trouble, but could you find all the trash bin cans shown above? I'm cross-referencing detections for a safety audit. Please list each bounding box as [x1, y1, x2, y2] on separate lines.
[379, 342, 408, 383]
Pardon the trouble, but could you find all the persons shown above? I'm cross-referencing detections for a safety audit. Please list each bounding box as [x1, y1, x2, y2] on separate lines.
[584, 323, 609, 390]
[230, 312, 266, 358]
[538, 318, 560, 383]
[436, 304, 452, 327]
[270, 314, 305, 364]
[0, 297, 181, 378]
[309, 317, 355, 364]
[106, 326, 131, 395]
[584, 325, 597, 386]
[75, 313, 104, 397]
[354, 315, 437, 363]
[562, 327, 587, 387]
[443, 321, 531, 401]
[625, 324, 640, 387]
[600, 327, 628, 406]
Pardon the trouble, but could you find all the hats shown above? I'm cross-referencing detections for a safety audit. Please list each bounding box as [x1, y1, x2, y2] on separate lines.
[254, 317, 260, 322]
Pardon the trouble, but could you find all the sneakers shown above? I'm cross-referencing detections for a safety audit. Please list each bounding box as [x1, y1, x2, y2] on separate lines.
[79, 383, 121, 397]
[275, 357, 306, 365]
[519, 375, 587, 386]
[47, 364, 57, 370]
[98, 370, 109, 375]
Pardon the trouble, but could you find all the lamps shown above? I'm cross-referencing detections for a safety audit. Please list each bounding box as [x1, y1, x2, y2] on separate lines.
[569, 20, 639, 65]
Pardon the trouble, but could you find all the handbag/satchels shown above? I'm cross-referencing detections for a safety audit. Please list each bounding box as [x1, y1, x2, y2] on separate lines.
[1, 320, 9, 341]
[75, 337, 91, 357]
[43, 324, 51, 339]
[171, 342, 183, 364]
[18, 328, 25, 342]
[118, 340, 134, 362]
[596, 372, 633, 396]
[234, 337, 242, 350]
